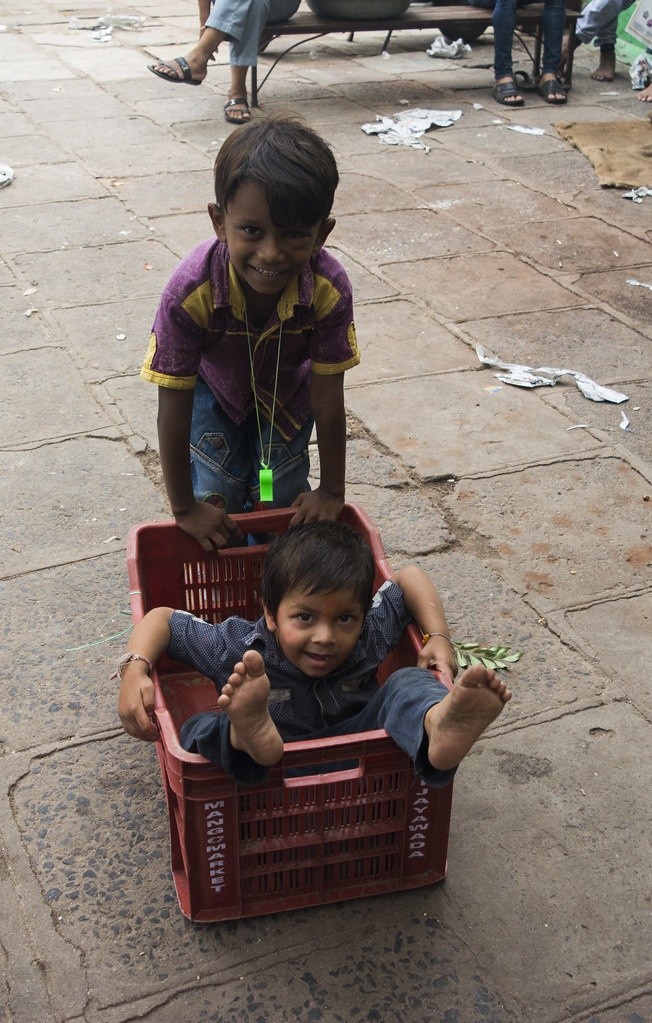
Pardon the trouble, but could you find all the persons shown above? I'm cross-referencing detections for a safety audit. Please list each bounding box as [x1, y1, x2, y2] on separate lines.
[466, 0, 568, 106]
[637, 83, 652, 102]
[141, 110, 362, 557]
[146, 0, 302, 122]
[559, 0, 636, 82]
[109, 520, 513, 789]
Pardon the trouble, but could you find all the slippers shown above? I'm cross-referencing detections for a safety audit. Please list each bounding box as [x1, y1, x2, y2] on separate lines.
[493, 81, 524, 107]
[513, 70, 538, 91]
[224, 98, 250, 124]
[556, 73, 571, 92]
[146, 56, 203, 86]
[536, 79, 567, 105]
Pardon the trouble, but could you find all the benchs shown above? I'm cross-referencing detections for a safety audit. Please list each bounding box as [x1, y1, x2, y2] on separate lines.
[212, 0, 584, 108]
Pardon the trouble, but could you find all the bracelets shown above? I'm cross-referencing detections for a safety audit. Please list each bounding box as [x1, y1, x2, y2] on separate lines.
[109, 653, 153, 681]
[421, 633, 454, 648]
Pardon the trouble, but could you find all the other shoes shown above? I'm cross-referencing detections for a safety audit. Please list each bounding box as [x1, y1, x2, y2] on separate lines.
[0, 164, 14, 188]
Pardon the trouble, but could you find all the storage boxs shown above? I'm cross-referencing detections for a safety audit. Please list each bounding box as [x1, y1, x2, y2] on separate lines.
[126, 504, 455, 926]
